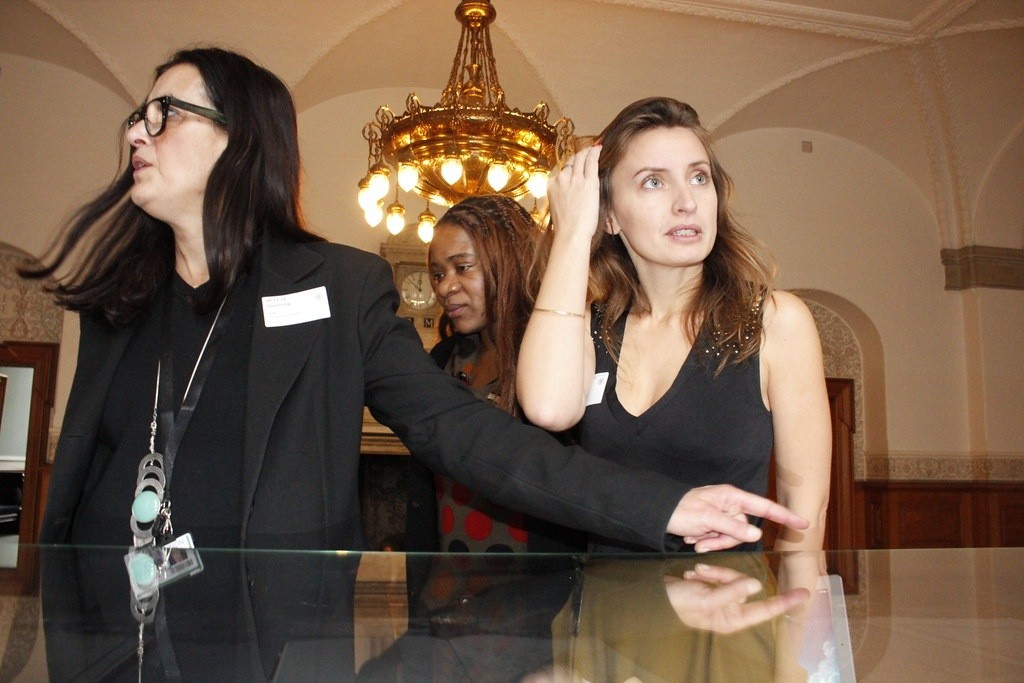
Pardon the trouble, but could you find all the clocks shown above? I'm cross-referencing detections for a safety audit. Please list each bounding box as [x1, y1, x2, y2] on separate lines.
[380, 224, 444, 350]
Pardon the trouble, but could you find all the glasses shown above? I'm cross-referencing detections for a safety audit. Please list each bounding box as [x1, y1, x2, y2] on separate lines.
[127, 95, 226, 137]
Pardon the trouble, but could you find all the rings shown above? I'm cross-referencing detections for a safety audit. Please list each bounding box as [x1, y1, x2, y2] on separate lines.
[561, 164, 573, 170]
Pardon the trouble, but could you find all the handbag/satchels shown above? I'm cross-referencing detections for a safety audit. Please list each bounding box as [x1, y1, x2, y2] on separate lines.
[430, 564, 576, 682]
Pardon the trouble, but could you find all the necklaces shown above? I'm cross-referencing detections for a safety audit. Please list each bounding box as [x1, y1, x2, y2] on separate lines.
[126, 295, 228, 546]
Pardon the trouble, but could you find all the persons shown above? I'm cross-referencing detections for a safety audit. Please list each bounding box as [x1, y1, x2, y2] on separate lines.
[354, 195, 575, 682]
[16, 46, 811, 683]
[166, 548, 188, 565]
[516, 97, 832, 683]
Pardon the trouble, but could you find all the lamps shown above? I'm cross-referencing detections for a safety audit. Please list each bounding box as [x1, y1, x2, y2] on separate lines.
[357, 0, 575, 244]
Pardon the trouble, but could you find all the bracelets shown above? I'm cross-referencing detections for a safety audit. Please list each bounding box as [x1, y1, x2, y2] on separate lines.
[533, 306, 586, 319]
